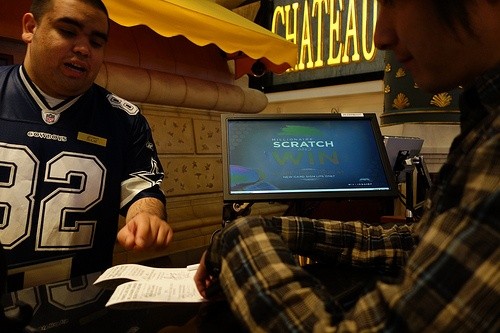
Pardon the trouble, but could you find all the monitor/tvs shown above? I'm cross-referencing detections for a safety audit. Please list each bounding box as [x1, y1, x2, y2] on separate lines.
[221, 112, 399, 202]
[384, 135, 424, 183]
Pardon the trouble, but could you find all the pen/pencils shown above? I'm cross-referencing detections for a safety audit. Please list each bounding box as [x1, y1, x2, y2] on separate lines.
[187, 263, 199, 271]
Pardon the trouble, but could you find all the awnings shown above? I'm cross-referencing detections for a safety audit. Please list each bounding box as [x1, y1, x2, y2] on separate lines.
[100, 0, 297, 79]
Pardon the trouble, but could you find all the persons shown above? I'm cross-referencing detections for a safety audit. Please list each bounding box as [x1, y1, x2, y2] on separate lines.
[0, 0, 174, 267]
[195, 0, 500, 333]
